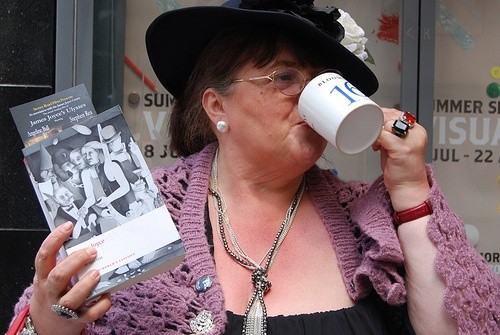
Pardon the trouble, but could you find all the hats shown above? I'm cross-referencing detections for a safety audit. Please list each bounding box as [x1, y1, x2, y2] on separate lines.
[101, 125, 121, 143]
[145, 0, 379, 101]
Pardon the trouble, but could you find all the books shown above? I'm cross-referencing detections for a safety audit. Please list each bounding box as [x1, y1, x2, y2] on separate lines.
[23, 105, 186, 307]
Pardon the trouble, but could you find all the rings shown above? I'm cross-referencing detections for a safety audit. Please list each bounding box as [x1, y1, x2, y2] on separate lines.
[50, 305, 79, 321]
[399, 112, 417, 129]
[391, 119, 409, 139]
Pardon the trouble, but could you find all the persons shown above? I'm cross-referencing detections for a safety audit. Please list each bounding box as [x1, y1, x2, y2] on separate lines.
[4, 0, 500, 335]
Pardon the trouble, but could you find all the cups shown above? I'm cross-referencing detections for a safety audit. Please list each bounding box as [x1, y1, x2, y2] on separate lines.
[298, 72, 384, 155]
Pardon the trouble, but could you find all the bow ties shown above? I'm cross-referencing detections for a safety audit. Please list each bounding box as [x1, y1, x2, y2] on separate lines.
[66, 205, 74, 212]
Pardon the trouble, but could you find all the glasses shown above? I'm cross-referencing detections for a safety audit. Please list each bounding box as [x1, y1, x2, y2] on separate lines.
[229, 68, 343, 96]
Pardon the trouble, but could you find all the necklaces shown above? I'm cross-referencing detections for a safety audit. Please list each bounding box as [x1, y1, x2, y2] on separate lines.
[208, 148, 307, 335]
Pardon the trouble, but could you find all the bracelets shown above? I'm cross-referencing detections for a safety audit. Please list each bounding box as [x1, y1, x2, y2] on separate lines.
[393, 198, 435, 226]
[22, 316, 37, 335]
